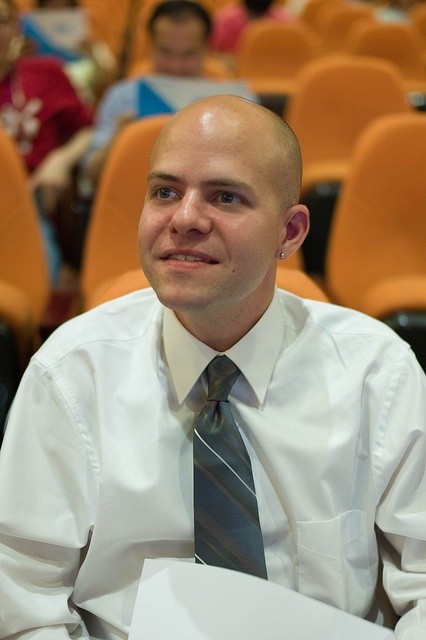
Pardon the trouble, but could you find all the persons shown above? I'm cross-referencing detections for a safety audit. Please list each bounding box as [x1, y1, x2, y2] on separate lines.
[0, 94, 426, 640]
[1, 1, 96, 324]
[77, 1, 260, 197]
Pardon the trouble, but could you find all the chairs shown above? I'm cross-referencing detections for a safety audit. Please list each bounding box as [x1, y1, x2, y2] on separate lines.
[239, 19, 317, 114]
[324, 113, 426, 322]
[317, 4, 371, 51]
[343, 17, 426, 94]
[298, 1, 328, 24]
[1, 126, 49, 345]
[284, 56, 412, 276]
[77, 114, 329, 312]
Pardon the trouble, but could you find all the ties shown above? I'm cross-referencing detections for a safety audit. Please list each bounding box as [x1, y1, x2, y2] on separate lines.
[193, 356, 268, 581]
